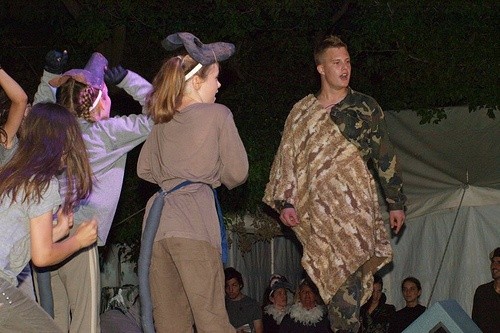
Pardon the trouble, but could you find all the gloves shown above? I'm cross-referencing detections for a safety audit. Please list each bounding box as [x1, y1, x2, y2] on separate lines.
[45, 49, 69, 74]
[103, 65, 128, 85]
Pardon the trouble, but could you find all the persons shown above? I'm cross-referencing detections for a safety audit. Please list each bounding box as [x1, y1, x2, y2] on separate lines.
[262, 274, 296, 333]
[261, 35, 407, 333]
[289, 277, 335, 333]
[223, 267, 264, 333]
[471, 246, 500, 333]
[136, 33, 251, 333]
[0, 101, 99, 333]
[16, 43, 154, 333]
[358, 276, 395, 333]
[390, 276, 427, 333]
[0, 66, 28, 167]
